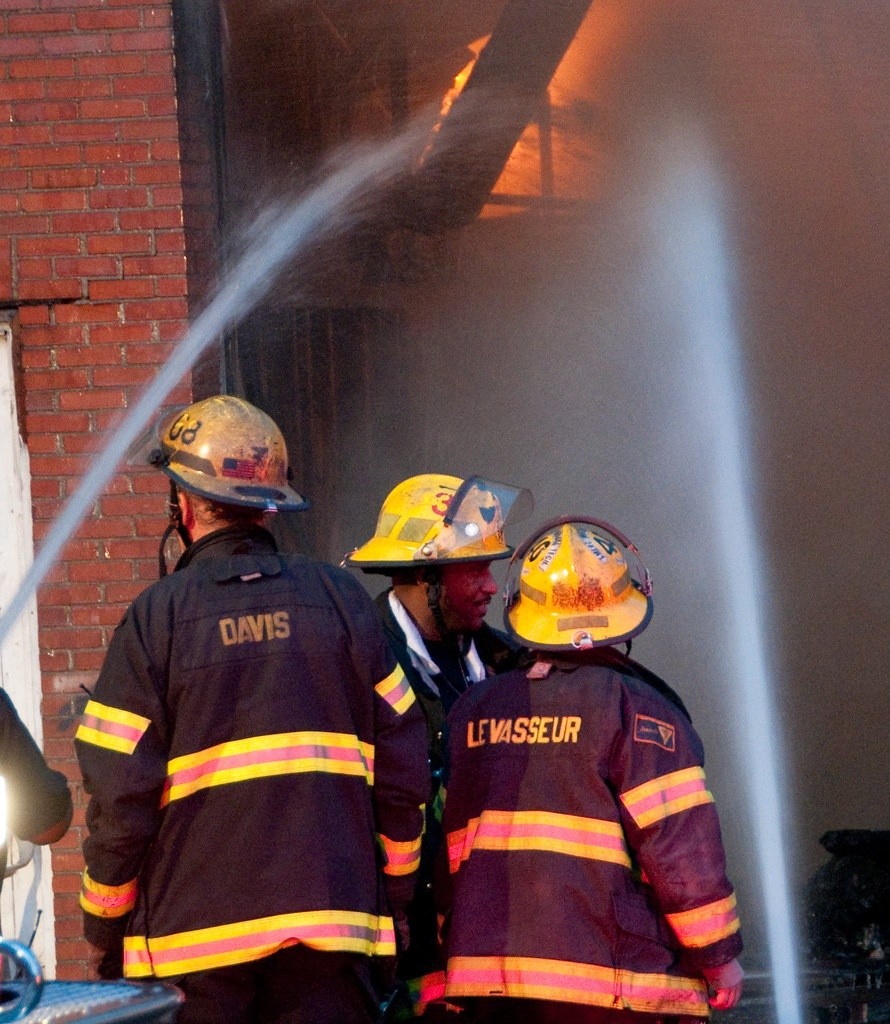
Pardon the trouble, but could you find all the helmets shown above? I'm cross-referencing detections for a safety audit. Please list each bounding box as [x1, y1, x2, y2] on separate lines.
[148, 397, 311, 511]
[499, 517, 651, 646]
[345, 475, 538, 568]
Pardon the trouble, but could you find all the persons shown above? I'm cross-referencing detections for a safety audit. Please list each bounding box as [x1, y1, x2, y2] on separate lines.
[343, 473, 521, 1022]
[83, 394, 434, 1024]
[0, 685, 77, 886]
[410, 514, 747, 1023]
